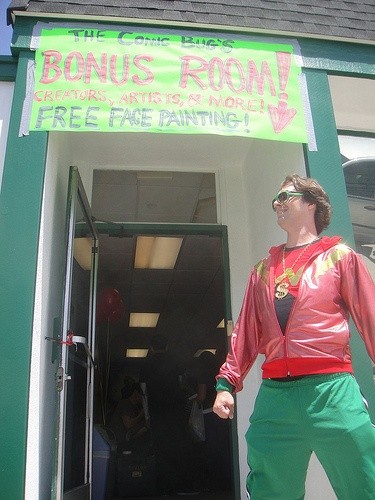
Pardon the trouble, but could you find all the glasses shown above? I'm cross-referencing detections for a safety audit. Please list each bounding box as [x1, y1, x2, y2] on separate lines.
[272, 190, 304, 212]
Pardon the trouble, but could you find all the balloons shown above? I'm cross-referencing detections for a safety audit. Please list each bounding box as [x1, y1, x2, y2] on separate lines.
[102, 287, 124, 321]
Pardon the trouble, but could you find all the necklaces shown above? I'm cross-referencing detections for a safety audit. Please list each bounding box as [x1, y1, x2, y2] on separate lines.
[275, 236, 319, 299]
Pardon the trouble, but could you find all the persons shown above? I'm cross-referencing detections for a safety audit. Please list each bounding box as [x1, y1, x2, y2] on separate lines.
[107, 355, 231, 492]
[213, 175, 375, 500]
